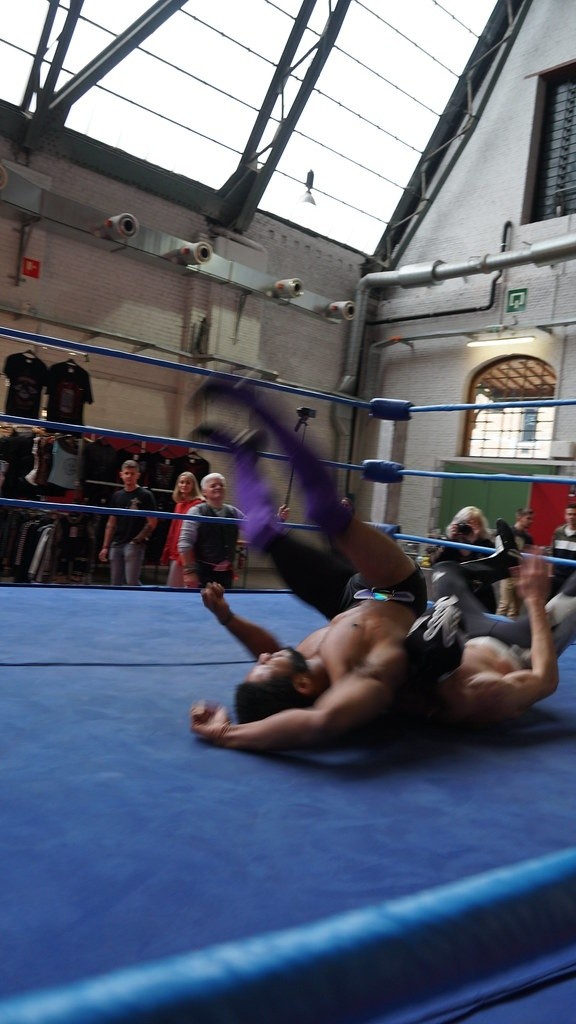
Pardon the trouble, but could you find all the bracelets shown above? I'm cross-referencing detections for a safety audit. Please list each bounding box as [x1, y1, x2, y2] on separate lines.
[132, 537, 139, 543]
[183, 568, 199, 574]
[219, 613, 235, 624]
[524, 596, 546, 605]
[102, 546, 109, 549]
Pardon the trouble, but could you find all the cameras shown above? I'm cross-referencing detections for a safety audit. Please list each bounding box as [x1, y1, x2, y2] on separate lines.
[295, 407, 317, 419]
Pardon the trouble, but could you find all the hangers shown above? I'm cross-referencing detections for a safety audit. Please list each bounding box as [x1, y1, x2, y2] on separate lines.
[99, 435, 110, 446]
[65, 353, 78, 365]
[15, 505, 72, 524]
[124, 441, 148, 453]
[1, 423, 75, 445]
[187, 447, 202, 460]
[22, 347, 37, 358]
[159, 445, 177, 457]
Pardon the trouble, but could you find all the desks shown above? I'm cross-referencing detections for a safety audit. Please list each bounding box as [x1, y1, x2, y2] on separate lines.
[230, 540, 251, 587]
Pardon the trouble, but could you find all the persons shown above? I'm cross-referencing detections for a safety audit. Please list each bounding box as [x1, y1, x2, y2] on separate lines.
[547, 503, 576, 604]
[182, 374, 575, 751]
[176, 471, 291, 590]
[160, 472, 207, 587]
[98, 459, 159, 586]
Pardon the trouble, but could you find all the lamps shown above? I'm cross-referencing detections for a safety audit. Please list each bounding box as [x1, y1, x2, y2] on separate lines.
[299, 171, 316, 205]
[467, 336, 535, 347]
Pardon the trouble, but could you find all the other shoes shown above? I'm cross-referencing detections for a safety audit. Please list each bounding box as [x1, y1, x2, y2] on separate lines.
[495, 518, 519, 551]
[186, 375, 266, 409]
[187, 420, 268, 454]
